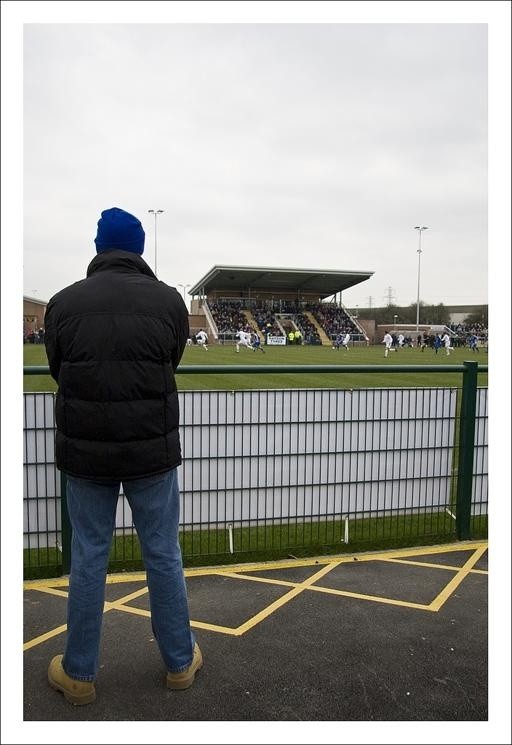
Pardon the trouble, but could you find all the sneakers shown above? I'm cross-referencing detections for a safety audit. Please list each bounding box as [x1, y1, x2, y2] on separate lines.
[165, 640, 204, 692]
[47, 653, 98, 707]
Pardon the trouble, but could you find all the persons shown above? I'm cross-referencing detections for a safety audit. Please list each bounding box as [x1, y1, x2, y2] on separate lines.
[44, 208, 202, 707]
[23, 327, 46, 345]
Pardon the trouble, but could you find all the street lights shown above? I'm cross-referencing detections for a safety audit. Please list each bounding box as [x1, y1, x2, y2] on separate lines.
[149, 210, 164, 276]
[415, 226, 428, 330]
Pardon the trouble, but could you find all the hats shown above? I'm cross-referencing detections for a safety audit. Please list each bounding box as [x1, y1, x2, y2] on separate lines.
[94, 206, 146, 255]
